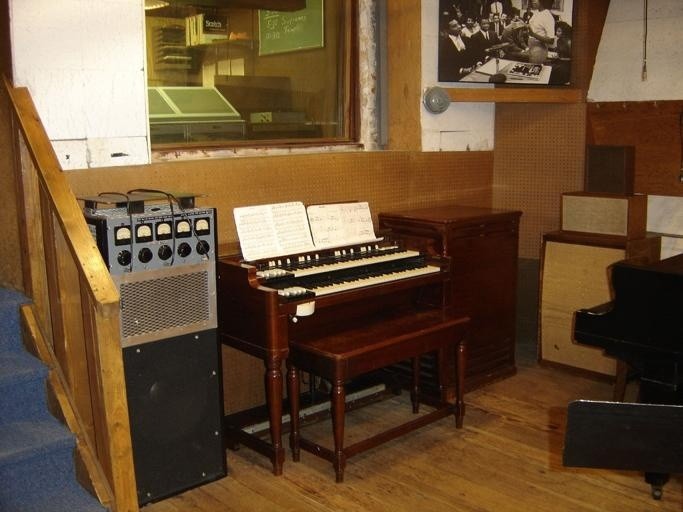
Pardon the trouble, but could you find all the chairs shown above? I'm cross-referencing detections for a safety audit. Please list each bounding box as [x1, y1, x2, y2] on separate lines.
[606, 254, 661, 402]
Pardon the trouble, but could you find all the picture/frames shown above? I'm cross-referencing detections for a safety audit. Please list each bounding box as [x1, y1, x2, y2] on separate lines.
[437, 0, 573, 86]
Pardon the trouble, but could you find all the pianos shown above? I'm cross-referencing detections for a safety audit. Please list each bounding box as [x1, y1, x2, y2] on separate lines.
[238, 235, 443, 304]
[571, 252, 683, 498]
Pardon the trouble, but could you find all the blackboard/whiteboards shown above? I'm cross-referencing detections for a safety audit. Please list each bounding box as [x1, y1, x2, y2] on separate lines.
[258, 0, 324, 57]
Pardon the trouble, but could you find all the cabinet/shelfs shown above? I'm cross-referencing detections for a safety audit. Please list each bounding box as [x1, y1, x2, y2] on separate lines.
[377, 204, 522, 402]
[535, 232, 662, 386]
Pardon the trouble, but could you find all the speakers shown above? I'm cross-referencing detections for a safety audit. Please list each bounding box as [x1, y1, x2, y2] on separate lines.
[73, 189, 229, 508]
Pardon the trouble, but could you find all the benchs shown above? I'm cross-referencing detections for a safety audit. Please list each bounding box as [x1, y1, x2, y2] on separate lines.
[284, 303, 469, 484]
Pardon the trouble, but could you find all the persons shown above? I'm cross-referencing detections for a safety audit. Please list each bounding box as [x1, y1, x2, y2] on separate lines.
[439, 1, 572, 85]
